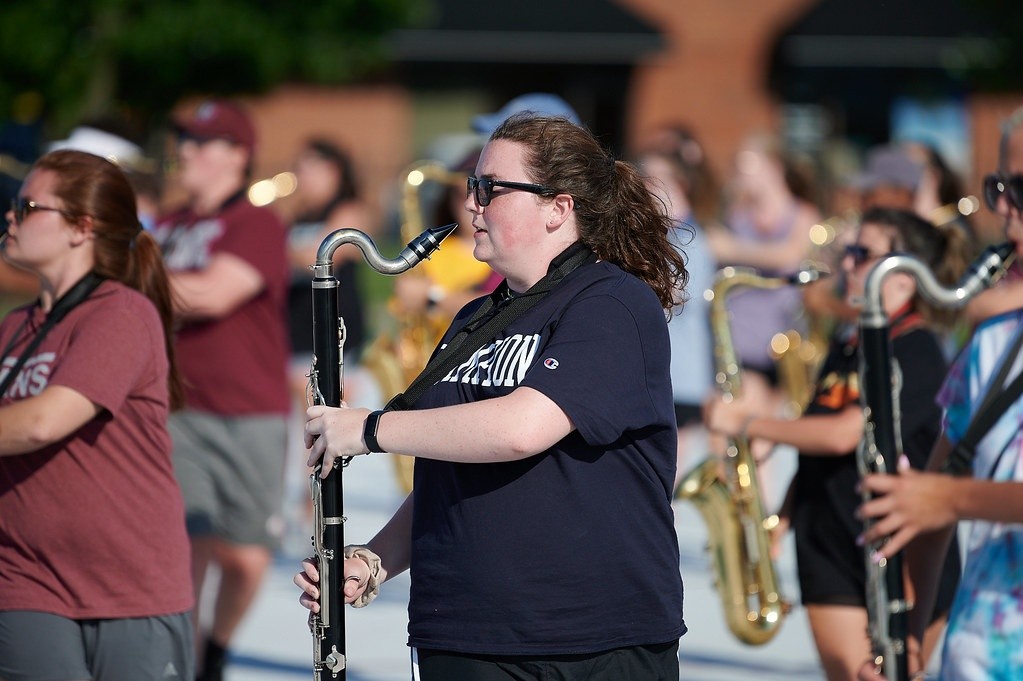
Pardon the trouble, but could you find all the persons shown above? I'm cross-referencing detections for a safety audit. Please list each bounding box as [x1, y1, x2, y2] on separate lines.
[0, 93, 1023, 681]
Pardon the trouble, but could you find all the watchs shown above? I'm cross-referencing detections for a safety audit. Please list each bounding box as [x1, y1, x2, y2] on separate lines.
[364, 410, 391, 453]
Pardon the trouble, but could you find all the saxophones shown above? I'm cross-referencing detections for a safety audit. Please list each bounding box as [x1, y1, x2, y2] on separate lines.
[358, 155, 467, 503]
[850, 234, 1018, 680]
[669, 261, 835, 651]
[302, 211, 461, 680]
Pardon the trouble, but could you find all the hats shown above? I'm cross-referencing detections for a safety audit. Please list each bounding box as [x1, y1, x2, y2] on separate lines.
[168, 102, 255, 146]
[471, 97, 581, 145]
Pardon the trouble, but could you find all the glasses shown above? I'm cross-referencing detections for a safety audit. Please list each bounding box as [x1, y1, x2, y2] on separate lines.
[466, 174, 581, 210]
[10, 197, 79, 225]
[984, 174, 1023, 213]
[847, 243, 896, 267]
